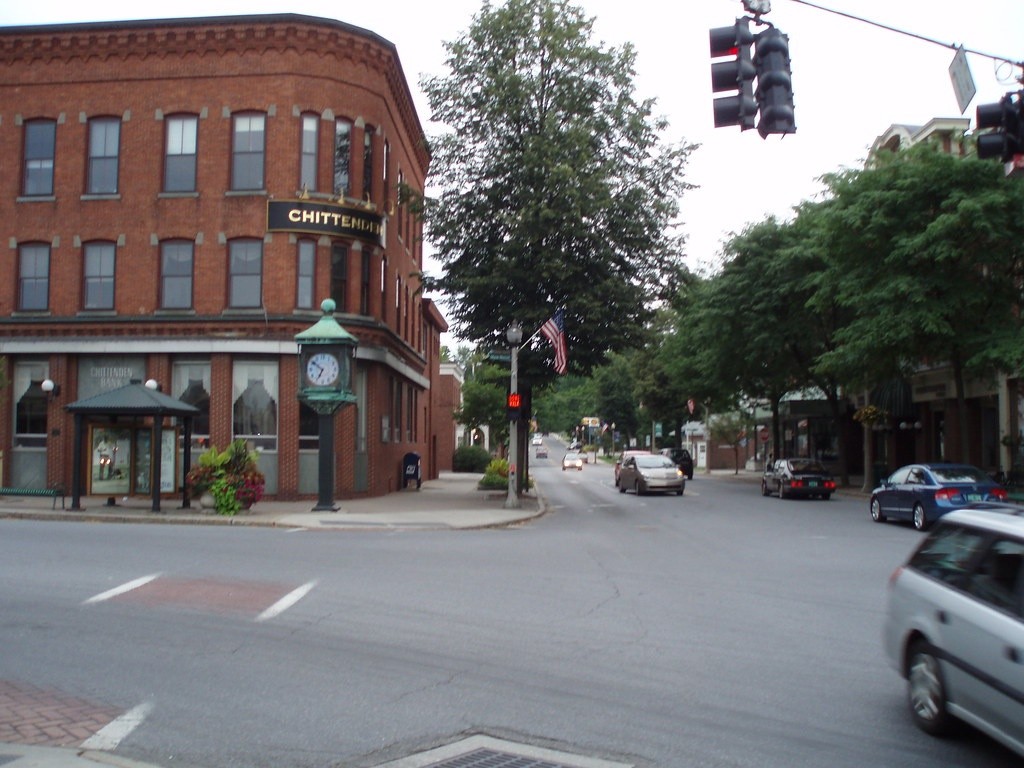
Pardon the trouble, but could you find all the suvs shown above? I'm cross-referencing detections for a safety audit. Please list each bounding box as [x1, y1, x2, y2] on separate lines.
[882, 499, 1024, 763]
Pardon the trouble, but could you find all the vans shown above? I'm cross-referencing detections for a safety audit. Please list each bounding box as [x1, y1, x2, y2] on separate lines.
[658, 448, 693, 480]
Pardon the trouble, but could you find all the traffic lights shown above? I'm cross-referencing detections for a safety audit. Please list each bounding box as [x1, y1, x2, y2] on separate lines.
[709, 16, 759, 132]
[507, 393, 522, 410]
[975, 91, 1016, 162]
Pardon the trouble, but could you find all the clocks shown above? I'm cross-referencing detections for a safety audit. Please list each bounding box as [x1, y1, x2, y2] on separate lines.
[294, 298, 359, 415]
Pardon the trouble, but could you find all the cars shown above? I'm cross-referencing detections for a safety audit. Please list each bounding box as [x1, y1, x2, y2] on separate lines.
[761, 456, 836, 501]
[614, 450, 653, 486]
[531, 436, 543, 445]
[567, 441, 588, 463]
[870, 462, 1010, 532]
[535, 446, 548, 459]
[618, 454, 685, 497]
[562, 452, 583, 470]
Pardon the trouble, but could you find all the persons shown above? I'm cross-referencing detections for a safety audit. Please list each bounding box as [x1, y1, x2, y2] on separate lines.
[97, 433, 113, 479]
[766, 453, 773, 472]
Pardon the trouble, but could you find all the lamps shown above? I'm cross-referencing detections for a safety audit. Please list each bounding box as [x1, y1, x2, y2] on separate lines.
[41, 379, 59, 396]
[914, 419, 923, 430]
[900, 419, 912, 432]
[145, 378, 161, 392]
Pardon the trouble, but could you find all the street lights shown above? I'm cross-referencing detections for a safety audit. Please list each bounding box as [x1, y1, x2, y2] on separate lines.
[504, 318, 523, 511]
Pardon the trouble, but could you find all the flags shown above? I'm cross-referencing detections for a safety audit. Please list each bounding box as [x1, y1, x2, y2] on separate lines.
[541, 310, 567, 375]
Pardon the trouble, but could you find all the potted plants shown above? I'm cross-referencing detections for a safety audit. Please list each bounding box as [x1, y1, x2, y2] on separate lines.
[185, 438, 265, 515]
[853, 405, 893, 431]
